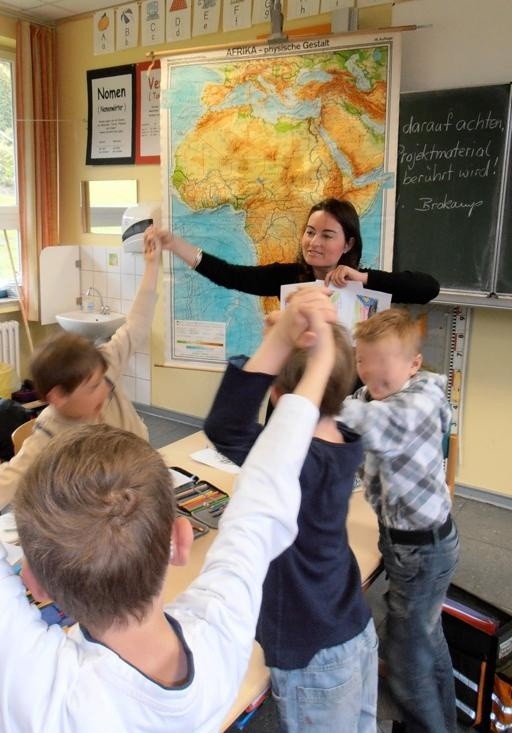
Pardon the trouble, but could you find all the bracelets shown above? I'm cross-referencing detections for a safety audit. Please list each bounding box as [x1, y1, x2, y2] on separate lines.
[190, 247, 202, 271]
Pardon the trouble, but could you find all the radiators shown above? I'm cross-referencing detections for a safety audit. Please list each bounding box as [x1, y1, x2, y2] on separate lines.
[0, 319, 20, 389]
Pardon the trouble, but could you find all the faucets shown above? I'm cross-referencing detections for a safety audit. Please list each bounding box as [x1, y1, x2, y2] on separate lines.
[85, 287, 110, 315]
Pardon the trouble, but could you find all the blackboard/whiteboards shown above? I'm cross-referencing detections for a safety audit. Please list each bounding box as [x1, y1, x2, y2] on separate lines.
[392, 82, 512, 310]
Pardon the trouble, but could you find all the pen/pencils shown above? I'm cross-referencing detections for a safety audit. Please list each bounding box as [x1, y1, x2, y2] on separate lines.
[175, 484, 230, 532]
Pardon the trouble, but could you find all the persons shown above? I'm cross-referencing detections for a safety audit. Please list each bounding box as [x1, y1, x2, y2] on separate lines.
[143, 197, 440, 426]
[0, 285, 335, 732]
[0, 222, 163, 516]
[333, 305, 460, 733]
[201, 286, 383, 732]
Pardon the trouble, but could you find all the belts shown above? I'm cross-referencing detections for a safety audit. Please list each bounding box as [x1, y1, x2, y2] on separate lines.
[375, 511, 454, 547]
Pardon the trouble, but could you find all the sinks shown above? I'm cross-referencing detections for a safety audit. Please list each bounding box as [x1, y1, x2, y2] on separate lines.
[55, 310, 127, 341]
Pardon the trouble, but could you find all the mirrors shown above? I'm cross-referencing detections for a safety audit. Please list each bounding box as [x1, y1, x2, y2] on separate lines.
[78, 177, 139, 236]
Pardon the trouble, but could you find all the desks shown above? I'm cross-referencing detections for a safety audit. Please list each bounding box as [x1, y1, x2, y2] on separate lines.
[0, 426, 385, 733]
